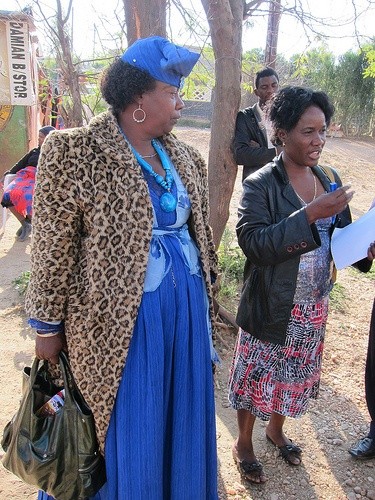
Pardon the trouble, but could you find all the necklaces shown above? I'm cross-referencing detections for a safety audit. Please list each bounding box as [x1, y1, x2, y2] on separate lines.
[141, 153, 160, 161]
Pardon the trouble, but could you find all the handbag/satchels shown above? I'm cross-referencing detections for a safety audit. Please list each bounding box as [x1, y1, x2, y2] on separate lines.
[1, 352, 106, 500]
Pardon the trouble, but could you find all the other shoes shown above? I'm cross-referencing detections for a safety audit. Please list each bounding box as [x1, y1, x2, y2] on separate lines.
[19, 224, 32, 241]
[16, 219, 31, 236]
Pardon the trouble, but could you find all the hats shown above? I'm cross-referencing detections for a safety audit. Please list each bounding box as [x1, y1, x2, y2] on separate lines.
[40, 126, 55, 136]
[122, 36, 202, 88]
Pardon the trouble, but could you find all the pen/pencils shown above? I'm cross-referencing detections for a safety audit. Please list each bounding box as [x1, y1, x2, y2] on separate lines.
[330, 183, 337, 224]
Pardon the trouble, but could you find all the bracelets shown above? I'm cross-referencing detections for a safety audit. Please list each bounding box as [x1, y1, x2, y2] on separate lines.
[31, 324, 68, 342]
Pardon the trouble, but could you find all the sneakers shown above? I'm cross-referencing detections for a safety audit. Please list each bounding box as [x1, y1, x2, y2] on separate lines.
[349, 433, 375, 459]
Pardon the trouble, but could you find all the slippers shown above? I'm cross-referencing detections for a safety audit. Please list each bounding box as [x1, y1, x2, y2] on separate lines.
[232, 446, 266, 485]
[266, 433, 302, 466]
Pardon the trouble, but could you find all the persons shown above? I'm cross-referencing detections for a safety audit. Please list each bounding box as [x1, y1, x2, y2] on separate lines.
[229, 67, 313, 188]
[234, 87, 375, 485]
[31, 34, 217, 500]
[0, 122, 58, 240]
[348, 286, 375, 465]
